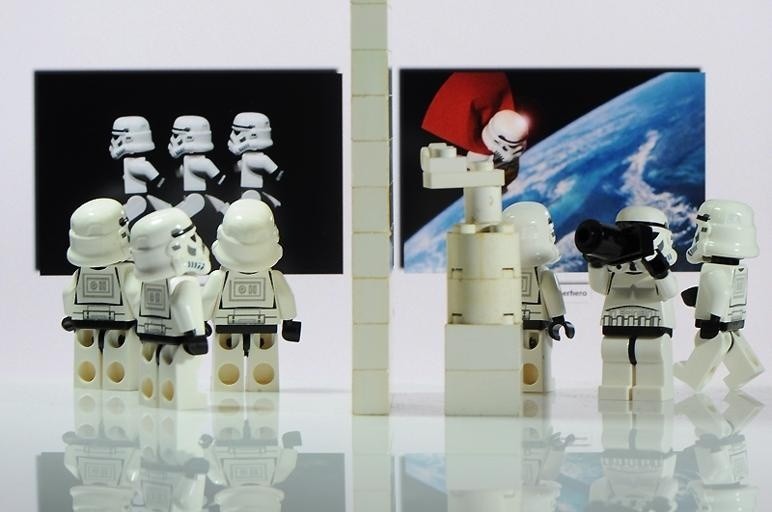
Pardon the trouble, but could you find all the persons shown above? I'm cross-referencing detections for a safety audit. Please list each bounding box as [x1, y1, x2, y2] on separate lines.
[61, 390, 145, 510]
[62, 196, 142, 391]
[107, 114, 176, 224]
[200, 197, 301, 393]
[670, 197, 768, 391]
[586, 399, 683, 512]
[129, 205, 213, 411]
[501, 201, 576, 395]
[196, 390, 305, 511]
[670, 390, 766, 511]
[475, 105, 534, 196]
[224, 112, 289, 214]
[518, 396, 574, 511]
[165, 111, 232, 233]
[134, 400, 214, 512]
[576, 204, 684, 402]
